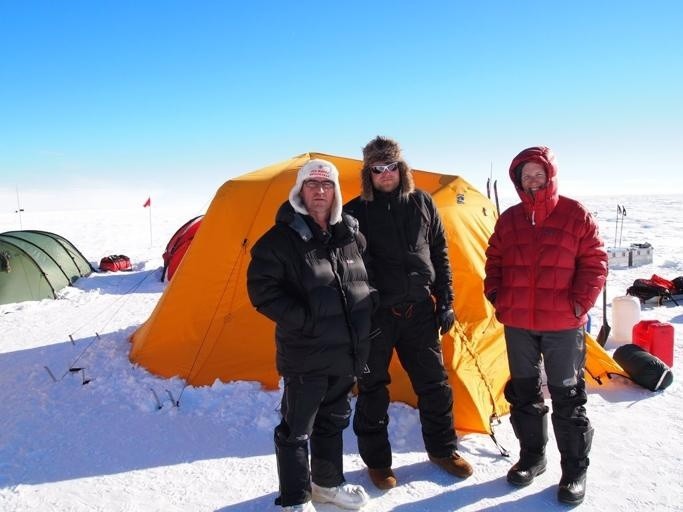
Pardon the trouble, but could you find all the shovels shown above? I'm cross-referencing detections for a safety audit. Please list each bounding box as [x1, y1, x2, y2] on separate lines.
[596, 280, 611, 348]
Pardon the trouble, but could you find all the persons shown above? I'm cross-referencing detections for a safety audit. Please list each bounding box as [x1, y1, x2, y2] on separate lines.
[247, 158, 379, 512]
[484, 146, 609, 506]
[343, 135, 474, 490]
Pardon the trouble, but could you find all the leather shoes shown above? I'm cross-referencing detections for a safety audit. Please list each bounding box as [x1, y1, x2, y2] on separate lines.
[507, 458, 547, 486]
[558, 473, 587, 504]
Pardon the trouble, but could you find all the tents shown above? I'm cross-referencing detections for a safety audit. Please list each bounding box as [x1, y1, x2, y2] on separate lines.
[0, 230, 98, 304]
[129, 152, 631, 440]
[100, 254, 132, 272]
[160, 215, 204, 283]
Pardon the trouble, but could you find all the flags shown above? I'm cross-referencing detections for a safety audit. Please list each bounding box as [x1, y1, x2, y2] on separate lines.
[623, 206, 626, 216]
[143, 197, 150, 207]
[617, 205, 622, 214]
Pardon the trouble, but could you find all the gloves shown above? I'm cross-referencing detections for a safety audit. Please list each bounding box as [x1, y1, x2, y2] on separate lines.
[438, 305, 454, 334]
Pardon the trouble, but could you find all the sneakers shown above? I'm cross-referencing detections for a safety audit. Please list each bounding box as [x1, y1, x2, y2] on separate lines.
[283, 468, 396, 512]
[429, 452, 473, 477]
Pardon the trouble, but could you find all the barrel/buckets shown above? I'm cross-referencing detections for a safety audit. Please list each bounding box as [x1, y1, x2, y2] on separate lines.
[648, 323, 674, 369]
[633, 320, 661, 353]
[612, 296, 640, 342]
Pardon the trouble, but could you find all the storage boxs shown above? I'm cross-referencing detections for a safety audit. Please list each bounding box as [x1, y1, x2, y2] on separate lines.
[626, 245, 653, 267]
[605, 245, 630, 269]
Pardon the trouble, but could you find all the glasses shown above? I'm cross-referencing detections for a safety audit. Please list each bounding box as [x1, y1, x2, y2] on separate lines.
[372, 162, 397, 173]
[304, 180, 334, 188]
